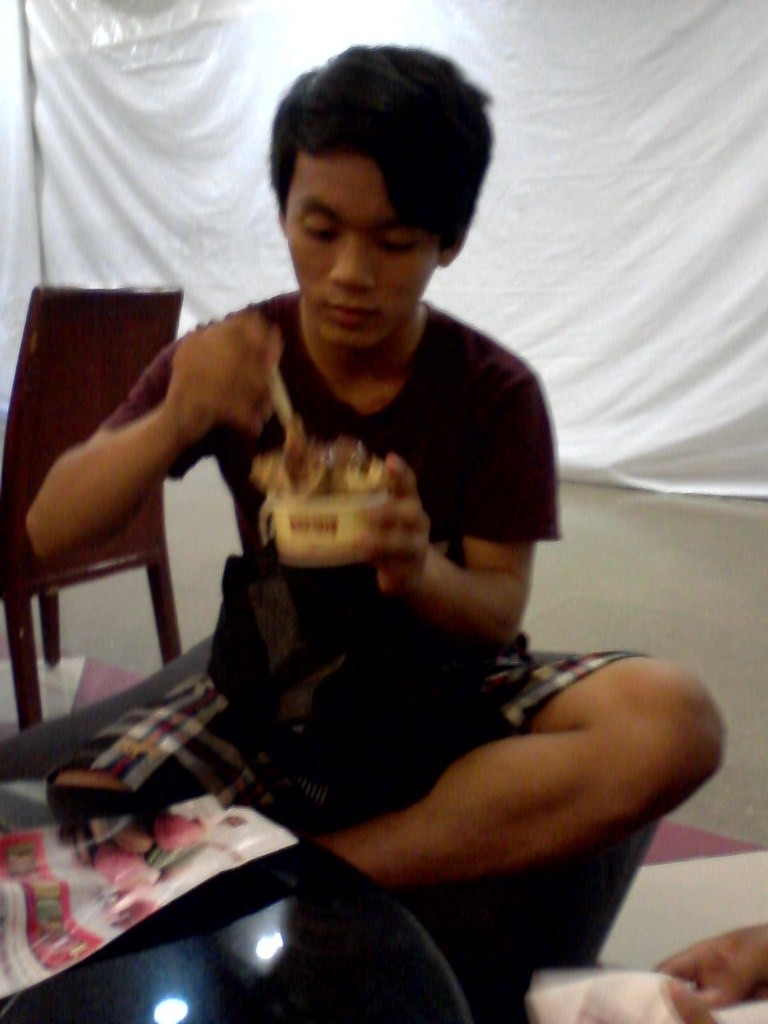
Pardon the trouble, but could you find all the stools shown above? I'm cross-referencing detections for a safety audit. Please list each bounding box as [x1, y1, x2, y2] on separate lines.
[0, 638, 658, 1024]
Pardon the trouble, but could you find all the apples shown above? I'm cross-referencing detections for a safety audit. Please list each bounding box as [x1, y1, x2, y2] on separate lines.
[94, 815, 206, 928]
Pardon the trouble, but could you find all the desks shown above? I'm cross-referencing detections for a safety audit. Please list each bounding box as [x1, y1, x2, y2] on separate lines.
[0, 830, 472, 1024]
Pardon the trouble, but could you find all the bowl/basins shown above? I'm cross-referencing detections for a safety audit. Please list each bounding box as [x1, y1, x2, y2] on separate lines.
[258, 490, 392, 567]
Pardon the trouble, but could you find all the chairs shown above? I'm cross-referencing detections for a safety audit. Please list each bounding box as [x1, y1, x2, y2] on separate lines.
[0, 286, 185, 730]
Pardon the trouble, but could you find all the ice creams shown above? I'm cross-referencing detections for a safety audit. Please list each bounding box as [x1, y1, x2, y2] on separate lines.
[251, 431, 392, 502]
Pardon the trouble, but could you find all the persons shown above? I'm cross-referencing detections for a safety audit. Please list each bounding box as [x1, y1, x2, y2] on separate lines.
[25, 40, 727, 883]
